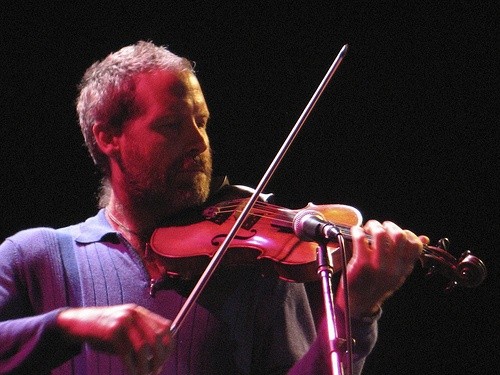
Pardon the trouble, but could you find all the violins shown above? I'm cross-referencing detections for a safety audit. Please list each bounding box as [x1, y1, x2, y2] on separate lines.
[147, 183, 489, 297]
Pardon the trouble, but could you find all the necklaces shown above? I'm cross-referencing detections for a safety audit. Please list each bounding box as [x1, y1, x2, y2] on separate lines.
[103, 206, 152, 244]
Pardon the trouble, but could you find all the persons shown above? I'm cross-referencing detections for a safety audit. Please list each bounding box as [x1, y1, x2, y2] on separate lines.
[1, 39, 434, 375]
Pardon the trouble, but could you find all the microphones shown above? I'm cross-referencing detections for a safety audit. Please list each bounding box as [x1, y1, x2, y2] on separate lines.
[292, 210, 343, 242]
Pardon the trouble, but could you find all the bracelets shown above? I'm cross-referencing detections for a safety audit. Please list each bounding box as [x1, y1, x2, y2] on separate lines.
[336, 303, 385, 327]
